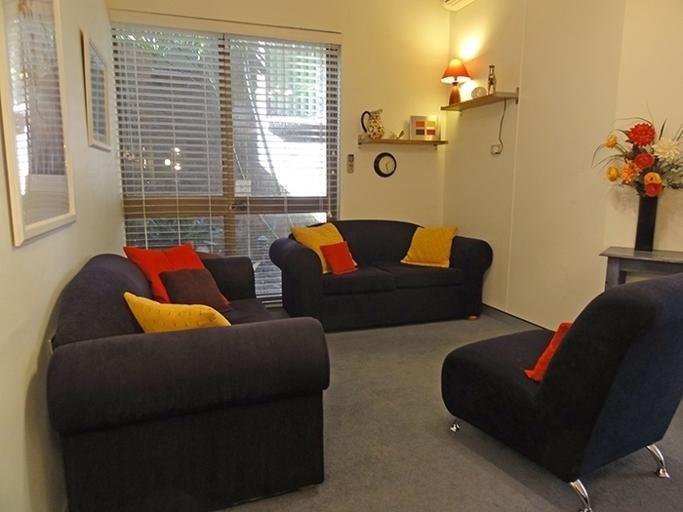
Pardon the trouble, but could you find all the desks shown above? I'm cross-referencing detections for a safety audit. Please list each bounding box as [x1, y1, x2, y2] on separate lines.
[597, 246, 682, 291]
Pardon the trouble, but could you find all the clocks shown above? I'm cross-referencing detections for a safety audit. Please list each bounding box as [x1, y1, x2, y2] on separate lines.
[374, 153, 396, 177]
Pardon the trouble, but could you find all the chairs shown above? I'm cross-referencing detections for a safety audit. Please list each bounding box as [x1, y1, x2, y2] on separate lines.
[441, 272, 683, 512]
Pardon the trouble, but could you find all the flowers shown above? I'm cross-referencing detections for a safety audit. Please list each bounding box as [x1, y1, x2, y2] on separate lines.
[591, 102, 683, 197]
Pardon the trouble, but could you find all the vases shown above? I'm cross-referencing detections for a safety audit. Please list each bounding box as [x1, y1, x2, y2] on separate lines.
[634, 190, 658, 252]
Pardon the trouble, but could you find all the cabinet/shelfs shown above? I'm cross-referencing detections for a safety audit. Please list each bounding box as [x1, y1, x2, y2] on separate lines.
[357, 88, 519, 149]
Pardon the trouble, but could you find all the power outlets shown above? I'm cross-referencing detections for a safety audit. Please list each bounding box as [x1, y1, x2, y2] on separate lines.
[491, 144, 501, 155]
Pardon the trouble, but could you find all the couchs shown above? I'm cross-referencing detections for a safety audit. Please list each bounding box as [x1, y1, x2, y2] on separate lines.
[46, 254, 330, 512]
[268, 220, 492, 333]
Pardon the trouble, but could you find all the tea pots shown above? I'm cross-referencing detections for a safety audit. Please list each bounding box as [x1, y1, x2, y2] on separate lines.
[359, 107, 385, 140]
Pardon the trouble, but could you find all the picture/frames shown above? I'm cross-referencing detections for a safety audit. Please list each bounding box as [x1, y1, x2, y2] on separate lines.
[82, 29, 112, 152]
[1, 0, 77, 248]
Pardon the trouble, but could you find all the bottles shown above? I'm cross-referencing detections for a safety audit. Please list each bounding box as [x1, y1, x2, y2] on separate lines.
[488, 64, 497, 95]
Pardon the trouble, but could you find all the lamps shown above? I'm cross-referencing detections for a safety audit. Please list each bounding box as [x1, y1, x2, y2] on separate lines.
[440, 58, 471, 106]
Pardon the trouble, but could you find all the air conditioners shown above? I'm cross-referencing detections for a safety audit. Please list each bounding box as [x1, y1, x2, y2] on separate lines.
[440, 0, 476, 10]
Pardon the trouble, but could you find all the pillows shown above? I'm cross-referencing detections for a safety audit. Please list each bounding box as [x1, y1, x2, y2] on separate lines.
[400, 225, 459, 268]
[289, 223, 358, 275]
[123, 291, 231, 334]
[159, 268, 234, 312]
[319, 241, 358, 277]
[123, 242, 231, 307]
[524, 321, 573, 382]
